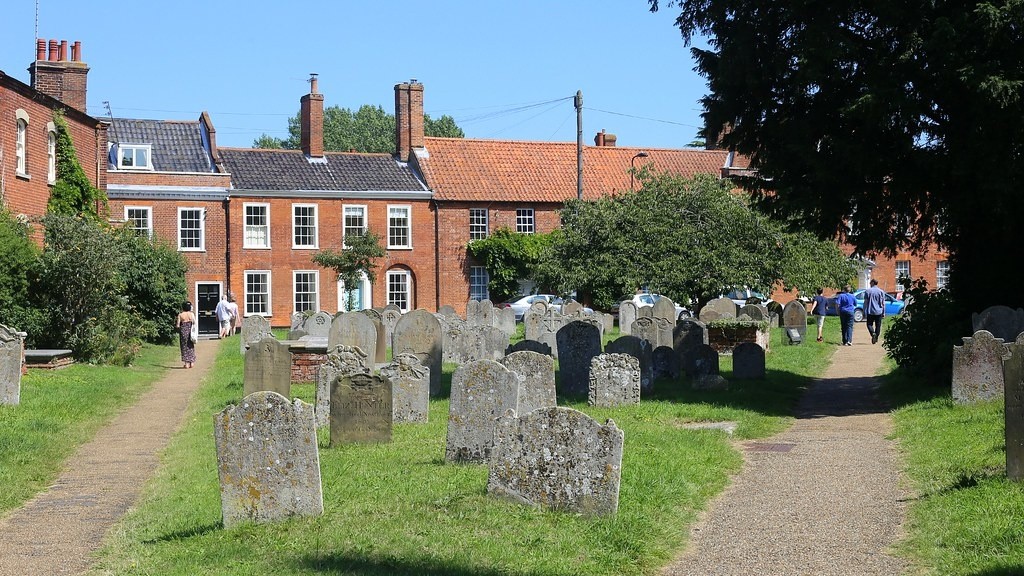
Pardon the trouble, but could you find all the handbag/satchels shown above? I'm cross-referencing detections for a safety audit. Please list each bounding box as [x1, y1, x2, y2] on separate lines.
[189, 325, 197, 344]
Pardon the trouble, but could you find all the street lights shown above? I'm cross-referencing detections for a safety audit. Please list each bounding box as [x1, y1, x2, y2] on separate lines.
[631, 150, 648, 189]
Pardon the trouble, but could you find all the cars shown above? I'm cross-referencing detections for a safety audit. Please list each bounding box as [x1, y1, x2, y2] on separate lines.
[717, 285, 786, 309]
[825, 288, 905, 322]
[610, 293, 691, 323]
[495, 294, 593, 322]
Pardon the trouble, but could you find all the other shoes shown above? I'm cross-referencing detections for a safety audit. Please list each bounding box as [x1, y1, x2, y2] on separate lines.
[842, 343, 847, 346]
[847, 341, 852, 346]
[190, 365, 193, 368]
[217, 336, 222, 339]
[872, 333, 878, 344]
[184, 364, 188, 369]
[818, 336, 823, 342]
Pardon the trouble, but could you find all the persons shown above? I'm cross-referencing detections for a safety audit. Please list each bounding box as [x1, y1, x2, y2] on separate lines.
[810, 287, 830, 342]
[863, 279, 885, 344]
[215, 295, 233, 339]
[176, 301, 198, 369]
[229, 296, 238, 336]
[836, 285, 857, 346]
